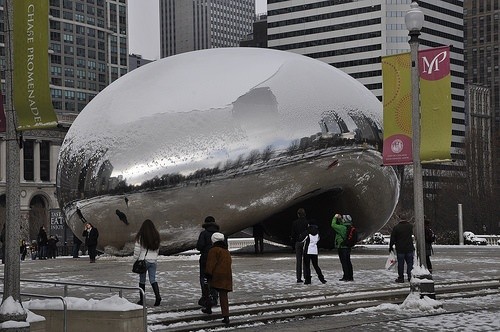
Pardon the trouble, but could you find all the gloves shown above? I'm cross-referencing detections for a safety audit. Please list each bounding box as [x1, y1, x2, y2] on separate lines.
[389, 247, 393, 252]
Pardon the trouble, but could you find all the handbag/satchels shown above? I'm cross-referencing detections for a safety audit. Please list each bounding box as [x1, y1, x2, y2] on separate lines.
[385, 251, 397, 268]
[132, 261, 147, 273]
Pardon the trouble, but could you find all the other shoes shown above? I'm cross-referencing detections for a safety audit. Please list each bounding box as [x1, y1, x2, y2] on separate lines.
[222, 317, 230, 324]
[395, 279, 404, 283]
[201, 309, 212, 314]
[304, 281, 311, 285]
[322, 280, 327, 283]
[297, 279, 303, 282]
[408, 278, 410, 282]
[254, 249, 263, 253]
[339, 278, 353, 282]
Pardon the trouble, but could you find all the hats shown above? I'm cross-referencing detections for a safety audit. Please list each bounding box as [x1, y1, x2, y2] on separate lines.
[343, 215, 352, 223]
[211, 233, 224, 243]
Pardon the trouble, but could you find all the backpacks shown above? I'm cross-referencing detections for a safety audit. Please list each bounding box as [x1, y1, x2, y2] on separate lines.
[424, 225, 435, 242]
[336, 225, 358, 247]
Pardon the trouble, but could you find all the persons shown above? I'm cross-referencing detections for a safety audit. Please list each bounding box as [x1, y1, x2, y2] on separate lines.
[325, 159, 339, 170]
[331, 213, 354, 281]
[196, 216, 233, 324]
[76, 207, 87, 223]
[253, 222, 269, 255]
[194, 179, 211, 187]
[0, 223, 6, 264]
[20, 226, 60, 260]
[71, 233, 82, 258]
[290, 208, 327, 284]
[82, 222, 99, 263]
[389, 213, 435, 283]
[125, 196, 129, 208]
[134, 219, 162, 307]
[116, 209, 130, 225]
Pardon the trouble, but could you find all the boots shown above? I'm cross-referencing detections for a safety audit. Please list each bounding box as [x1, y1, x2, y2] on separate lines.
[151, 281, 161, 306]
[137, 283, 146, 305]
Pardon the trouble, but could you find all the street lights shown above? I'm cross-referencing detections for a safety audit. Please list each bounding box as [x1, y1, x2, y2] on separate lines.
[404, 1, 436, 301]
